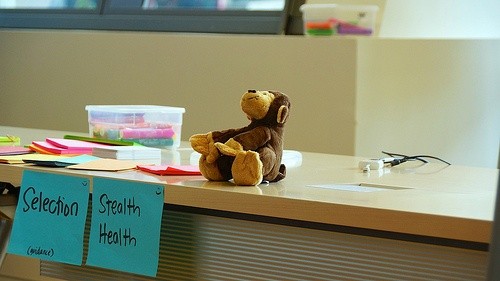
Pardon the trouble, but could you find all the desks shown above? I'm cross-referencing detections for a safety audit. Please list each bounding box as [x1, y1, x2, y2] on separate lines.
[0, 125, 498, 281]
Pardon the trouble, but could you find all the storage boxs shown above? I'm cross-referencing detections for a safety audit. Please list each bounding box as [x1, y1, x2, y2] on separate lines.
[298, 2, 380, 36]
[83, 103, 186, 150]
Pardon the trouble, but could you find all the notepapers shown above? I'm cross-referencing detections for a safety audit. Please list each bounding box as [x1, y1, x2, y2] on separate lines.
[0, 135, 203, 175]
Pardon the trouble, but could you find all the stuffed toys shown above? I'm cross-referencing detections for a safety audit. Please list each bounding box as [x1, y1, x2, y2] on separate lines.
[189, 90, 291, 186]
